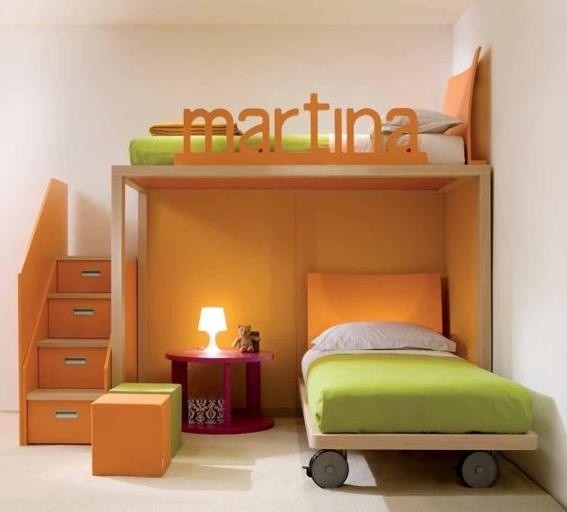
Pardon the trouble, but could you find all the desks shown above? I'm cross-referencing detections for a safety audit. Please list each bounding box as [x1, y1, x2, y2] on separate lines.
[163, 350, 275, 435]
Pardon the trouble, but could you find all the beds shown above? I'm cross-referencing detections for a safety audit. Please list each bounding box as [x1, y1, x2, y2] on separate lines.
[297, 268, 541, 491]
[128, 44, 491, 165]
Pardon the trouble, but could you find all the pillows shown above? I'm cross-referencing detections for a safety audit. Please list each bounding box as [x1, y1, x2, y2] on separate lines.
[372, 108, 462, 133]
[310, 323, 458, 353]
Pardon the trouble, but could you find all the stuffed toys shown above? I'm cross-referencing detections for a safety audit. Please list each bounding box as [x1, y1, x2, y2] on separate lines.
[233, 322, 260, 353]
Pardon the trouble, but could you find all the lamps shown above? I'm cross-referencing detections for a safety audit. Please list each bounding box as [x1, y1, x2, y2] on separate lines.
[196, 306, 229, 353]
